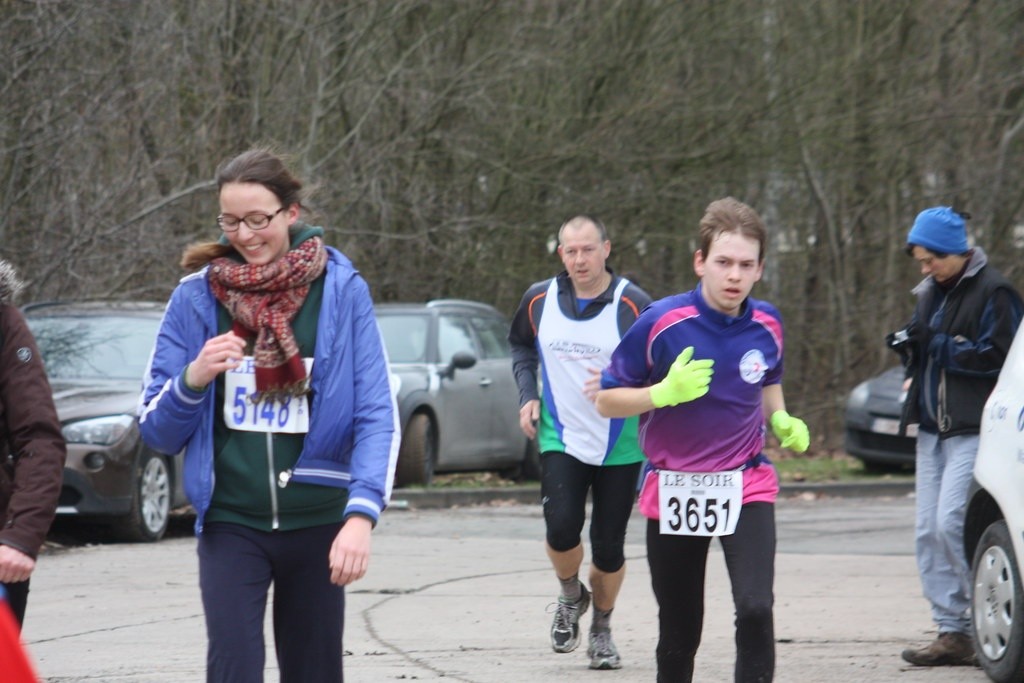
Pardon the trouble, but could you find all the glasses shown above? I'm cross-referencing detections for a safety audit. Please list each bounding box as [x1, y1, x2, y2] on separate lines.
[216, 207, 284, 233]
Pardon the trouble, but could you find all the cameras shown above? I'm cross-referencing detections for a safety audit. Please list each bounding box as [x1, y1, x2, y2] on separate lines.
[894, 329, 910, 343]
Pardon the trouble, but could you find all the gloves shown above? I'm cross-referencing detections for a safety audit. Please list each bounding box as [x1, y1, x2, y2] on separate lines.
[770, 409, 809, 457]
[886, 322, 932, 353]
[648, 346, 715, 409]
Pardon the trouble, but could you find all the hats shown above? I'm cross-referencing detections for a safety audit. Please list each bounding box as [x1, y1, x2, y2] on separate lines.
[907, 207, 972, 254]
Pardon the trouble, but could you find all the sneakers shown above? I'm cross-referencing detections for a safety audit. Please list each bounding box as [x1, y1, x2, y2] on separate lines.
[545, 580, 590, 652]
[587, 629, 621, 670]
[902, 632, 979, 666]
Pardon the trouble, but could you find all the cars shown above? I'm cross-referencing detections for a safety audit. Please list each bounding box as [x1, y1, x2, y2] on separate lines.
[958, 307, 1024, 683]
[842, 365, 919, 477]
[19, 294, 197, 546]
[369, 299, 543, 488]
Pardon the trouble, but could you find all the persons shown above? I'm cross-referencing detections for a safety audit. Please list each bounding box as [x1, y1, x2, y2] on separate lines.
[135, 147, 410, 682]
[884, 203, 1024, 667]
[0, 255, 69, 636]
[597, 195, 810, 683]
[507, 212, 650, 670]
